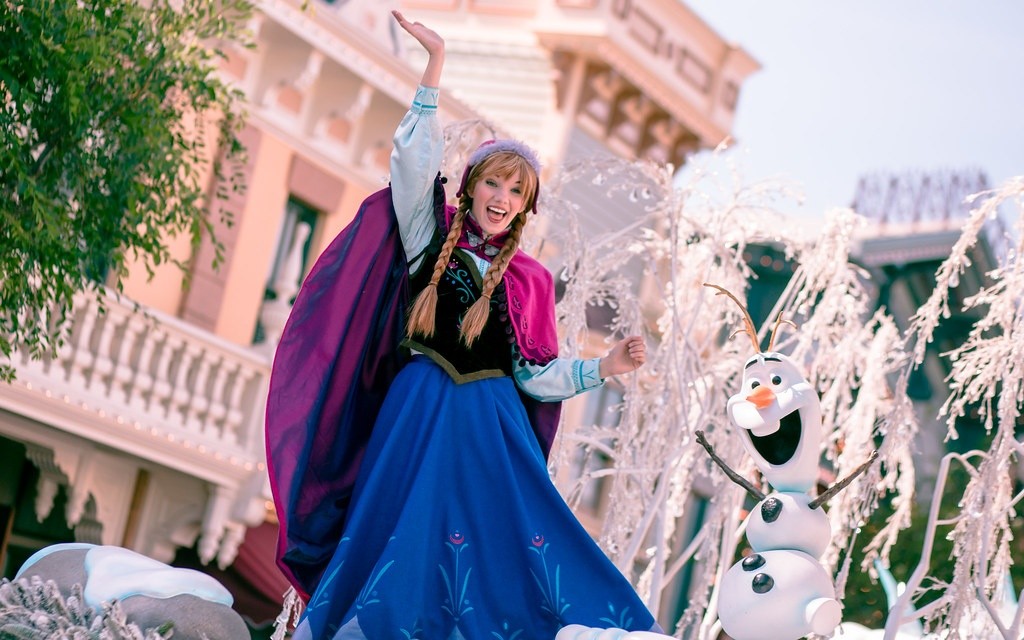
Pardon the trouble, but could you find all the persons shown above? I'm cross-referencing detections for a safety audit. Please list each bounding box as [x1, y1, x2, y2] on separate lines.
[274, 8, 661, 640]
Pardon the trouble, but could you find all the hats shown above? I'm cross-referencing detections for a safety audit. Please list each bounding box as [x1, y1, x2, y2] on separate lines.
[456, 139, 544, 214]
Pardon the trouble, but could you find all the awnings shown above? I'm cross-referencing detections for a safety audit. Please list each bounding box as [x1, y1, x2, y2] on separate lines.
[167, 517, 307, 638]
